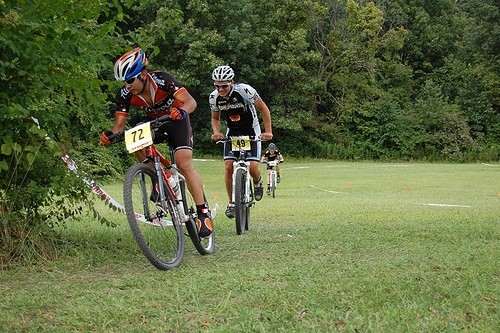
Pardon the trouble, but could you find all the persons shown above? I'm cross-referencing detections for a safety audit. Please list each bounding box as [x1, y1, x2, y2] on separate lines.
[208, 65, 273, 218]
[262, 142, 284, 197]
[99, 47, 214, 239]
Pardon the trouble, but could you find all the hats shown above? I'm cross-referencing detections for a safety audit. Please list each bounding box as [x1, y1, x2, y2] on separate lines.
[211, 65, 235, 82]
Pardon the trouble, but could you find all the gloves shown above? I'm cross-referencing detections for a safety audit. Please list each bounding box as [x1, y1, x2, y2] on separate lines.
[169, 107, 187, 121]
[100, 130, 117, 146]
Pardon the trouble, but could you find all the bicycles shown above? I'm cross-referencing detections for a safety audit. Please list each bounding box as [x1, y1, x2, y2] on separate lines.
[103, 112, 216, 271]
[263, 161, 281, 198]
[211, 136, 267, 235]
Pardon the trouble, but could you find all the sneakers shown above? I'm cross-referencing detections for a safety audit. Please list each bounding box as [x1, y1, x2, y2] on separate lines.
[225, 199, 236, 219]
[148, 183, 162, 202]
[195, 202, 213, 236]
[253, 176, 264, 201]
[276, 175, 280, 182]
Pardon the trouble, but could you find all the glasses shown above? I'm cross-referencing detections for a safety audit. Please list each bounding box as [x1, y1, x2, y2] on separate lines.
[111, 73, 142, 86]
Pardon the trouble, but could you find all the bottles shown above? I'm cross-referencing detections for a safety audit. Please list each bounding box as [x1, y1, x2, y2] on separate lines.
[164, 170, 180, 196]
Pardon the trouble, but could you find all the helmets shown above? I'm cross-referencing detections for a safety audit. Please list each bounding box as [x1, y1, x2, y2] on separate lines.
[269, 142, 275, 149]
[114, 47, 148, 82]
